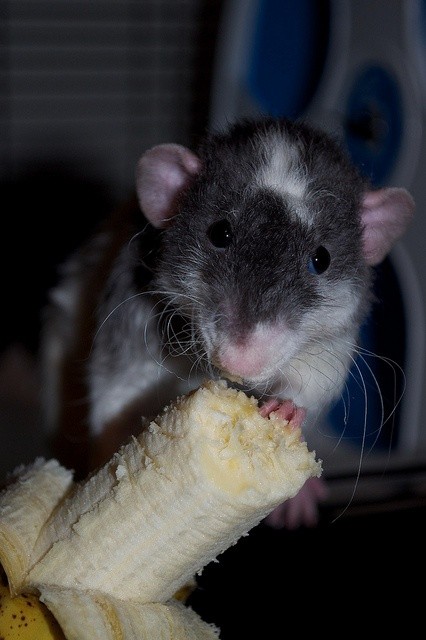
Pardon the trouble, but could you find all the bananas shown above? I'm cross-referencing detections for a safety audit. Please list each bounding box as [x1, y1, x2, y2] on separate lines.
[0, 379, 322, 639]
